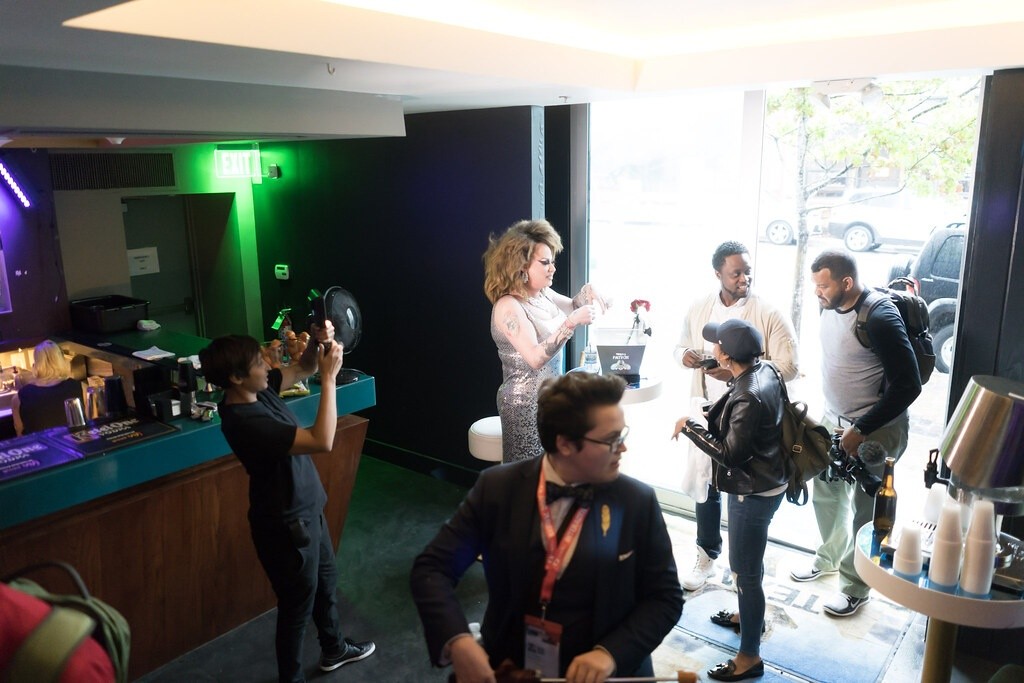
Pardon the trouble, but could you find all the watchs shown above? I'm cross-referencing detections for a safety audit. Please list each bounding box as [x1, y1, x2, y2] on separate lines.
[850, 422, 863, 436]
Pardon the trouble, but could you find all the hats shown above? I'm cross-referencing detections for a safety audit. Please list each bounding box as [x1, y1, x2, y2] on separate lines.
[701, 318, 765, 359]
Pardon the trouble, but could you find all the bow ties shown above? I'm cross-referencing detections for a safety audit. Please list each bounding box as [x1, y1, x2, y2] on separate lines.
[545, 480, 593, 510]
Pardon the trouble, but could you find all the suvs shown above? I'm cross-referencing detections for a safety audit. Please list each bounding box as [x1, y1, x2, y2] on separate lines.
[883, 220, 968, 377]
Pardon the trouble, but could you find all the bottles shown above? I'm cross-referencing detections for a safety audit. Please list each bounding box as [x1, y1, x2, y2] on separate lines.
[873, 456, 897, 535]
[275, 306, 291, 356]
[469, 622, 484, 648]
[581, 351, 600, 376]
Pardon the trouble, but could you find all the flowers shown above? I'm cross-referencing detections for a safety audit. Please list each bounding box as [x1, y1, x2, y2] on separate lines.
[630, 298, 652, 338]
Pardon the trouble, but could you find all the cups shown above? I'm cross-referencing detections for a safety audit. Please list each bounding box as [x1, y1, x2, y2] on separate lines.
[892, 499, 998, 594]
[179, 361, 198, 392]
[64, 375, 128, 429]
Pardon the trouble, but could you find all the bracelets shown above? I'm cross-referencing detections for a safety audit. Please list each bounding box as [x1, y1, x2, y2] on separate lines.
[570, 319, 578, 325]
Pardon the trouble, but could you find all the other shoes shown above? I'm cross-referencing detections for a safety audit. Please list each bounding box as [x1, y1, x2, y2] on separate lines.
[707, 658, 764, 682]
[711, 610, 765, 633]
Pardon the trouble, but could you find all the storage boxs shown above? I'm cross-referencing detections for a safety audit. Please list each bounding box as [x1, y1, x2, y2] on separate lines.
[69, 294, 150, 335]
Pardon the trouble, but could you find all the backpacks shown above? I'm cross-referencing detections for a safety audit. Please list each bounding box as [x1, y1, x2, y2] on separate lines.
[857, 277, 936, 385]
[764, 360, 833, 506]
[5, 557, 130, 683]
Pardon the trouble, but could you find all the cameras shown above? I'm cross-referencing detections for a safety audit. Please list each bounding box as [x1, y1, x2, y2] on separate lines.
[829, 435, 882, 497]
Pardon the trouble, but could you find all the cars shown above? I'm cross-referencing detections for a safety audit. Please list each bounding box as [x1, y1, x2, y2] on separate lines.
[765, 164, 960, 256]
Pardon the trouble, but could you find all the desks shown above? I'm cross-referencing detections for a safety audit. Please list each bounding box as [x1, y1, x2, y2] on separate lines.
[855, 519, 1024, 683]
[620, 376, 658, 403]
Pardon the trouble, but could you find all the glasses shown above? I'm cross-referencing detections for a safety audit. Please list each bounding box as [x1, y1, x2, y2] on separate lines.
[579, 425, 629, 453]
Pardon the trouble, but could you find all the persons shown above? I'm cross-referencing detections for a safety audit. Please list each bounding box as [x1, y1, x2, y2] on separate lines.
[10, 340, 90, 437]
[483, 219, 612, 465]
[674, 240, 799, 591]
[790, 251, 922, 617]
[0, 584, 115, 683]
[410, 373, 685, 682]
[671, 320, 790, 682]
[199, 320, 376, 683]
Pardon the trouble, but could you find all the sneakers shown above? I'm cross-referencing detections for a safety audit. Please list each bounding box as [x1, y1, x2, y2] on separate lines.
[823, 593, 870, 616]
[790, 564, 839, 582]
[681, 545, 716, 590]
[318, 639, 376, 671]
[732, 572, 739, 593]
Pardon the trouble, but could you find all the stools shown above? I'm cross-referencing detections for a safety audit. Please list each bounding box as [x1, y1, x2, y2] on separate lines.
[468, 416, 503, 465]
